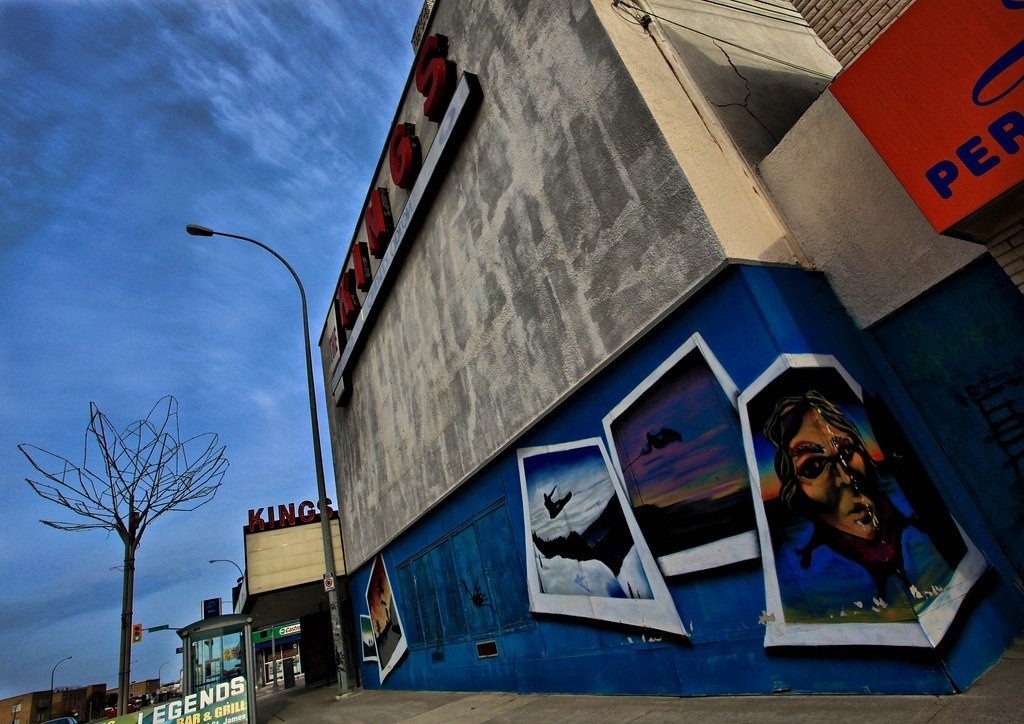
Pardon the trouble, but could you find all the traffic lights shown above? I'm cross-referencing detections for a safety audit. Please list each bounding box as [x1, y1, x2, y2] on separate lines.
[132, 623, 142, 644]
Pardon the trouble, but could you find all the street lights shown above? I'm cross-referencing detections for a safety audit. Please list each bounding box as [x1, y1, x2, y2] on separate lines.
[185, 222, 350, 697]
[49, 655, 72, 720]
[159, 661, 170, 688]
[208, 559, 245, 579]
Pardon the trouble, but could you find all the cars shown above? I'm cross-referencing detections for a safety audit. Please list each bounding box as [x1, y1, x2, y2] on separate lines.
[107, 698, 138, 719]
[39, 716, 77, 724]
